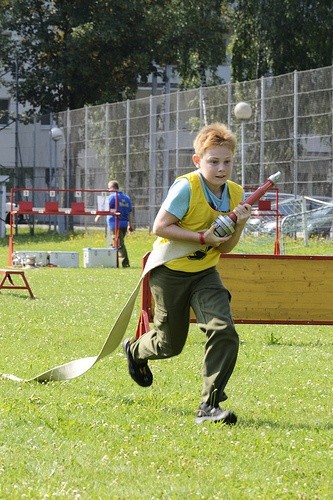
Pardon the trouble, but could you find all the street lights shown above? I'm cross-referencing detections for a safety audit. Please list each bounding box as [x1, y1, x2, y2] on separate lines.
[234, 101, 253, 187]
[49, 126, 64, 190]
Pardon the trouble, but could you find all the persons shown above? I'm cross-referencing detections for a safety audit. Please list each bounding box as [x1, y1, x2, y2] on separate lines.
[121, 122, 252, 425]
[94, 180, 134, 267]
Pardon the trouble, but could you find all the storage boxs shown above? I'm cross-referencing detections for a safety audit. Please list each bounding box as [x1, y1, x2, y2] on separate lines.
[15, 251, 48, 267]
[48, 251, 80, 268]
[82, 248, 118, 268]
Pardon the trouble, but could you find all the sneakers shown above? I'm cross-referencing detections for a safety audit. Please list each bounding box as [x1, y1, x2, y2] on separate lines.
[123, 339, 153, 387]
[196, 405, 236, 424]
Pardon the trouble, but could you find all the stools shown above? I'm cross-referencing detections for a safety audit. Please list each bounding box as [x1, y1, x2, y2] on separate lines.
[0, 269, 35, 298]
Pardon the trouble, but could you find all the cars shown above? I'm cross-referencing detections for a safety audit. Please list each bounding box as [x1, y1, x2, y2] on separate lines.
[241, 191, 333, 241]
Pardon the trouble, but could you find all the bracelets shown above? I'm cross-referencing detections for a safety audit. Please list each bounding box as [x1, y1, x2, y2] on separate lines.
[199, 232, 206, 245]
